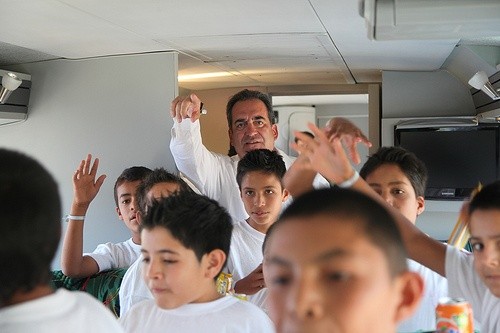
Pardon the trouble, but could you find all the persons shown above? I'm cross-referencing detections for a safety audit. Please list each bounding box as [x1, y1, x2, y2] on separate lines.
[290, 122, 500, 333]
[281, 116, 448, 333]
[169, 89, 332, 224]
[117, 188, 277, 333]
[61, 154, 152, 278]
[227, 149, 289, 312]
[0, 147, 126, 333]
[134, 167, 188, 224]
[261, 187, 426, 333]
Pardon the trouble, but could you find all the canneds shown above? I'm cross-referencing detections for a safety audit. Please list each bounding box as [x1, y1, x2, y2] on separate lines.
[233, 294, 247, 301]
[433, 298, 475, 333]
[215, 272, 233, 294]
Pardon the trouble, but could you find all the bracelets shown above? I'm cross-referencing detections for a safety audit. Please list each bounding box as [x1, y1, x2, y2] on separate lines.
[335, 169, 359, 188]
[65, 215, 85, 221]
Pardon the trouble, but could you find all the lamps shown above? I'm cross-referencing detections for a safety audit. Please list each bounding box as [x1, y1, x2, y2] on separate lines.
[467, 70, 500, 101]
[0, 71, 22, 104]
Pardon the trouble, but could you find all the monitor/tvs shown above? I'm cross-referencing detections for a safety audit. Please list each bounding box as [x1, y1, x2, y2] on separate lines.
[394, 116, 500, 201]
